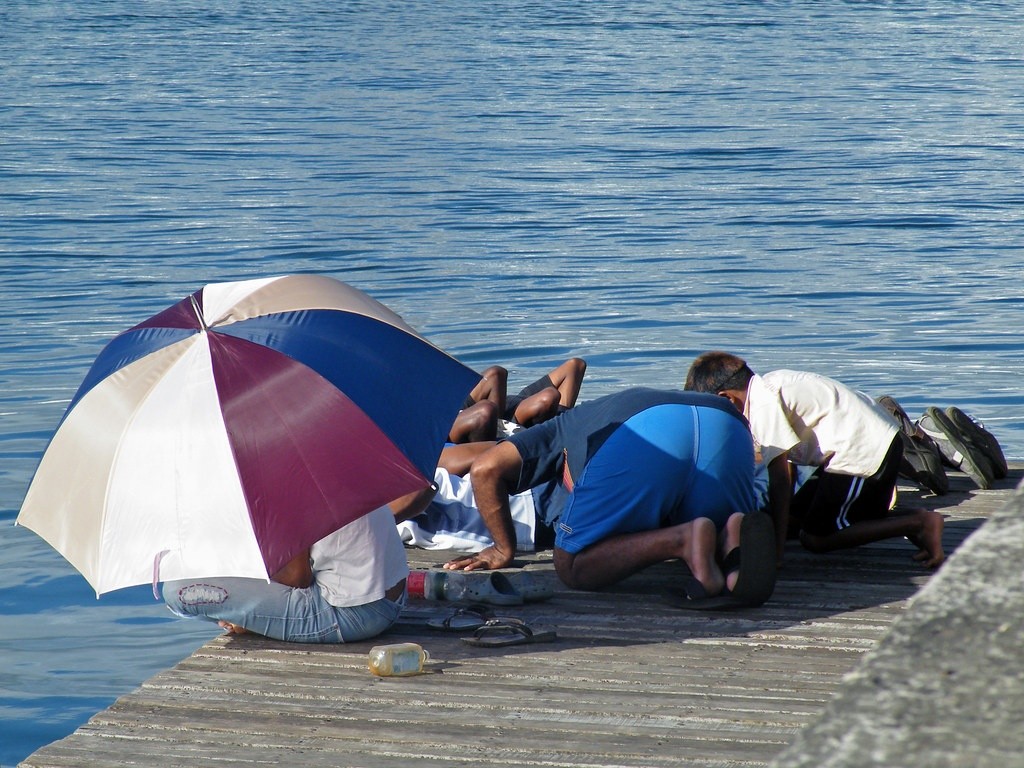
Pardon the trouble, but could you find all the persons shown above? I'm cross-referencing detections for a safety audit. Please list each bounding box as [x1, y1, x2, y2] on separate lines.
[163, 481, 438, 643]
[388, 359, 587, 554]
[443, 385, 777, 609]
[681, 352, 1009, 569]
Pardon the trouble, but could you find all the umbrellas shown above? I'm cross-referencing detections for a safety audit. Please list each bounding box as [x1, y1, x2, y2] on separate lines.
[14, 274, 487, 599]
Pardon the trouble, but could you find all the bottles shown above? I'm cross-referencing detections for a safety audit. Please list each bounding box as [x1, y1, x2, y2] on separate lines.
[368, 643, 430, 676]
[406, 571, 467, 602]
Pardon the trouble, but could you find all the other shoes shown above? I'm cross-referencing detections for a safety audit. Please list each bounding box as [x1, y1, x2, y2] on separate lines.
[918, 407, 994, 490]
[945, 406, 1008, 480]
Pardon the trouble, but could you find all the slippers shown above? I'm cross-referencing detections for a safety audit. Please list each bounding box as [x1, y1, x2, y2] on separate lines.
[460, 618, 557, 647]
[719, 511, 776, 609]
[507, 571, 554, 599]
[426, 609, 489, 631]
[664, 577, 734, 609]
[467, 571, 523, 605]
